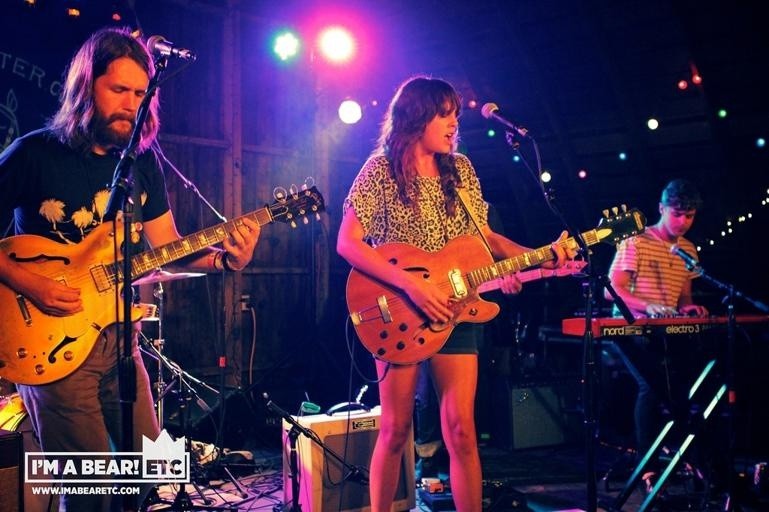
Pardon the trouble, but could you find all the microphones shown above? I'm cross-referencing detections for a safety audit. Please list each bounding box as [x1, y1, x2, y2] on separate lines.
[481, 102, 528, 139]
[147, 35, 195, 61]
[670, 243, 698, 268]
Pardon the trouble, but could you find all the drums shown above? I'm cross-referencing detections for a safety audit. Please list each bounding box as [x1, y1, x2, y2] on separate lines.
[0, 391, 122, 512]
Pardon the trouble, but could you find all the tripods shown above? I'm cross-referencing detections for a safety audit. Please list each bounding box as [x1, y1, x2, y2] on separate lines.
[106, 65, 282, 508]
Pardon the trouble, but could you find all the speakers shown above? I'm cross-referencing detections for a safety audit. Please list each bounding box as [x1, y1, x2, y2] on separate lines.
[282, 406, 416, 512]
[511, 384, 581, 449]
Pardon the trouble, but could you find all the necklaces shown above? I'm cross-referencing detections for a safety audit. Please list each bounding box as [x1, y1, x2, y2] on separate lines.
[415, 167, 449, 244]
[657, 229, 679, 268]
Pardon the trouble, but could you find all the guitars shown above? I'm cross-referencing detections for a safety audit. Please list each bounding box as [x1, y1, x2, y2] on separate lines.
[0, 176, 325, 387]
[346, 204, 648, 366]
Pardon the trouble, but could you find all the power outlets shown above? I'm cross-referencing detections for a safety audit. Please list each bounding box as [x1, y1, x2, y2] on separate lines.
[242, 294, 250, 311]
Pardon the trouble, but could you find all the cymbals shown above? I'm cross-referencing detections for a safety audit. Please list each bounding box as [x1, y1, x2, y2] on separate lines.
[130, 268, 207, 286]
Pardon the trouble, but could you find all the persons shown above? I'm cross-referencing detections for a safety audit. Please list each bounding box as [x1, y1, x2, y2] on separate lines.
[604, 180, 709, 499]
[336, 75, 567, 512]
[0, 27, 261, 512]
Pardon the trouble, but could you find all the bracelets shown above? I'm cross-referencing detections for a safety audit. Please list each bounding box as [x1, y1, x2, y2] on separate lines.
[208, 249, 246, 274]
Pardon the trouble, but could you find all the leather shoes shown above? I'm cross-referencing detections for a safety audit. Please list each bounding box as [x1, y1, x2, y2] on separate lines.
[645, 471, 670, 507]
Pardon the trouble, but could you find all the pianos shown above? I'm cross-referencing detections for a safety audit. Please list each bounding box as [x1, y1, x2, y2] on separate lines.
[562, 313, 768, 337]
